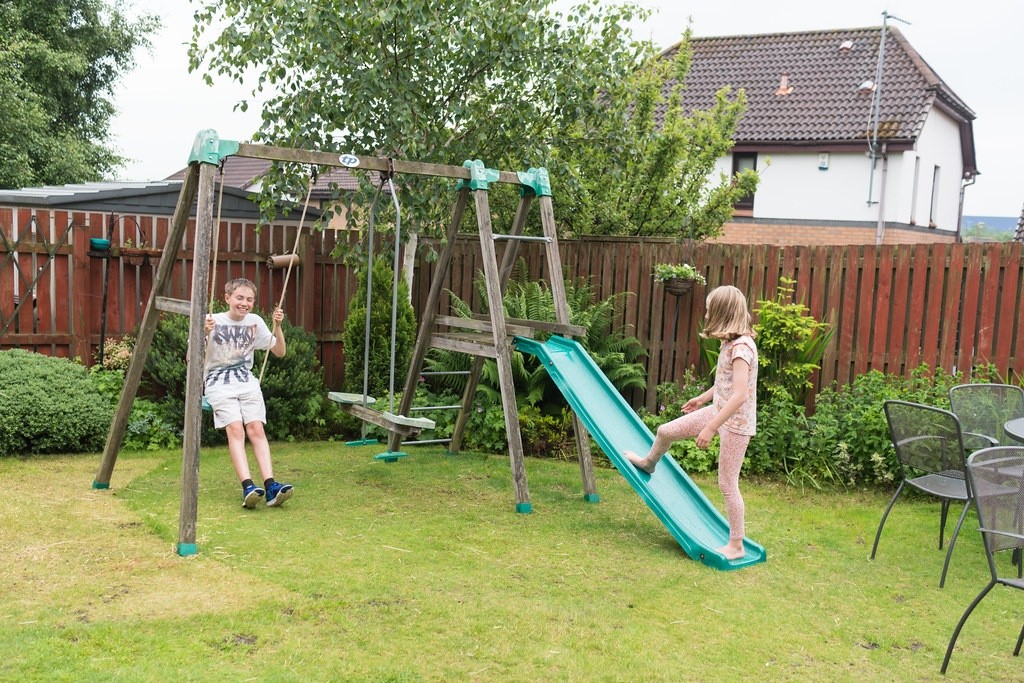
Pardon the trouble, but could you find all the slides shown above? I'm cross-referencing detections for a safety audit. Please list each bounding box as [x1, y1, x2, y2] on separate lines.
[504, 333, 770, 568]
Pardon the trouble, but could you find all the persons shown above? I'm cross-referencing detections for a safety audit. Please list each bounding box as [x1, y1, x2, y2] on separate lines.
[623, 286, 758, 560]
[186, 278, 294, 509]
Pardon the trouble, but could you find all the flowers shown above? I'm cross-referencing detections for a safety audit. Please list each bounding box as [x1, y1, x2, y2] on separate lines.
[650, 262, 707, 289]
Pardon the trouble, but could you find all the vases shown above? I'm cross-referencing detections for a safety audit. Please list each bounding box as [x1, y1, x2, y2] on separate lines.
[662, 276, 696, 297]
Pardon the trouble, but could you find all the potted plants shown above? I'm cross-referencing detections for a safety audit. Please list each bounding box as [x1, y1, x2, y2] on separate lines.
[119, 238, 162, 267]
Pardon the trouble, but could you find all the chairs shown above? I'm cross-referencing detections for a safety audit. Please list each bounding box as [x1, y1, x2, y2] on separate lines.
[938, 447, 1024, 675]
[870, 398, 1017, 587]
[947, 384, 1024, 555]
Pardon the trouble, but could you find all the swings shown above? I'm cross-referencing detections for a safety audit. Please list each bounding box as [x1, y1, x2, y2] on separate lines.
[203, 156, 314, 413]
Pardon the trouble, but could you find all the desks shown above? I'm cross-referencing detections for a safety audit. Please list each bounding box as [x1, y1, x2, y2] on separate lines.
[1004, 416, 1024, 579]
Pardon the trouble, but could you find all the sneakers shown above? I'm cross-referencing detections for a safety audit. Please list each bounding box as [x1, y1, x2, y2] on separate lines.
[265, 482, 294, 506]
[242, 484, 265, 509]
